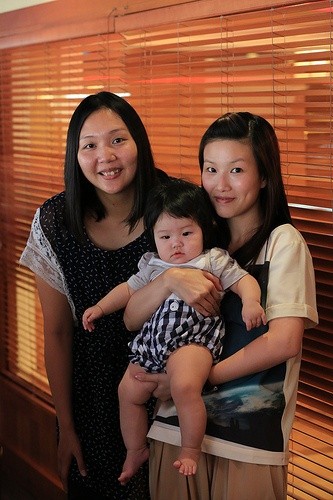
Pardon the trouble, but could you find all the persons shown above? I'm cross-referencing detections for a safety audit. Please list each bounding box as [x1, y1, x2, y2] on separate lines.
[20, 91, 200, 500]
[82, 183, 267, 485]
[150, 111, 318, 499]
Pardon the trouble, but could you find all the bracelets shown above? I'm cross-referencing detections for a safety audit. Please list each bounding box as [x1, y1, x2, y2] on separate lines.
[97, 304, 105, 314]
[203, 379, 217, 394]
